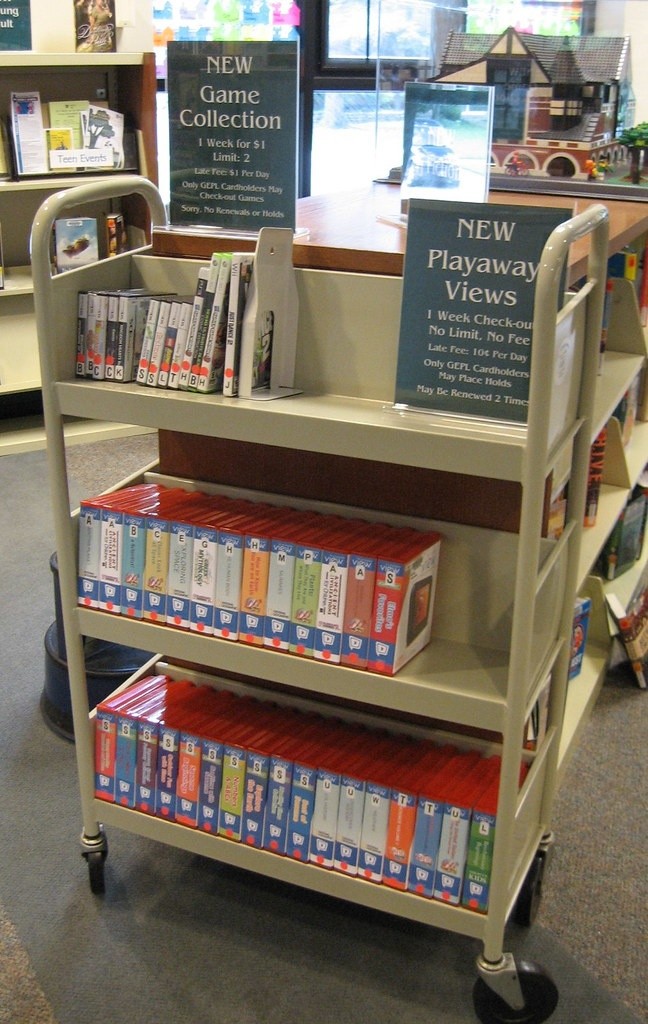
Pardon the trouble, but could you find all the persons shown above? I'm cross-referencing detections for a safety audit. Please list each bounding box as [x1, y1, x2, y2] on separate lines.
[511, 151, 519, 176]
[89, 0, 112, 51]
[584, 157, 606, 180]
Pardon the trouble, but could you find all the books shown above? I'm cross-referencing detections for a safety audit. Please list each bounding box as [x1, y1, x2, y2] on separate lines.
[106, 212, 129, 258]
[51, 216, 98, 274]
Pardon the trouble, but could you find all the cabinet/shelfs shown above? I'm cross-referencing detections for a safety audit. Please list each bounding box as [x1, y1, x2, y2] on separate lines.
[29, 174, 648, 1024]
[0, 51, 160, 457]
